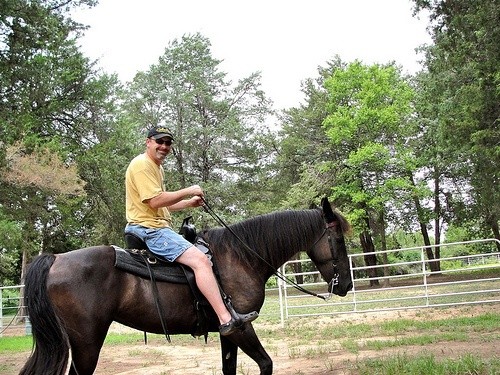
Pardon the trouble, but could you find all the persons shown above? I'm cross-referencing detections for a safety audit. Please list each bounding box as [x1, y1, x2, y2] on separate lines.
[125, 125, 260, 335]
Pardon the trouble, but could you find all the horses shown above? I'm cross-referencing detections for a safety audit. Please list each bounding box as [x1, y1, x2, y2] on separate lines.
[19, 196, 355, 375]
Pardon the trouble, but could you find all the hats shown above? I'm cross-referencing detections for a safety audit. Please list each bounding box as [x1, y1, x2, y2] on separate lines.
[148, 125, 174, 141]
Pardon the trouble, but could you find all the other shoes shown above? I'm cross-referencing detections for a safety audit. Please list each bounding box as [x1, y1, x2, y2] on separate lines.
[218, 311, 258, 335]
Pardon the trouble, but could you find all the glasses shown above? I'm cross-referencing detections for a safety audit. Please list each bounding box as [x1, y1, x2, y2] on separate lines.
[150, 138, 172, 146]
